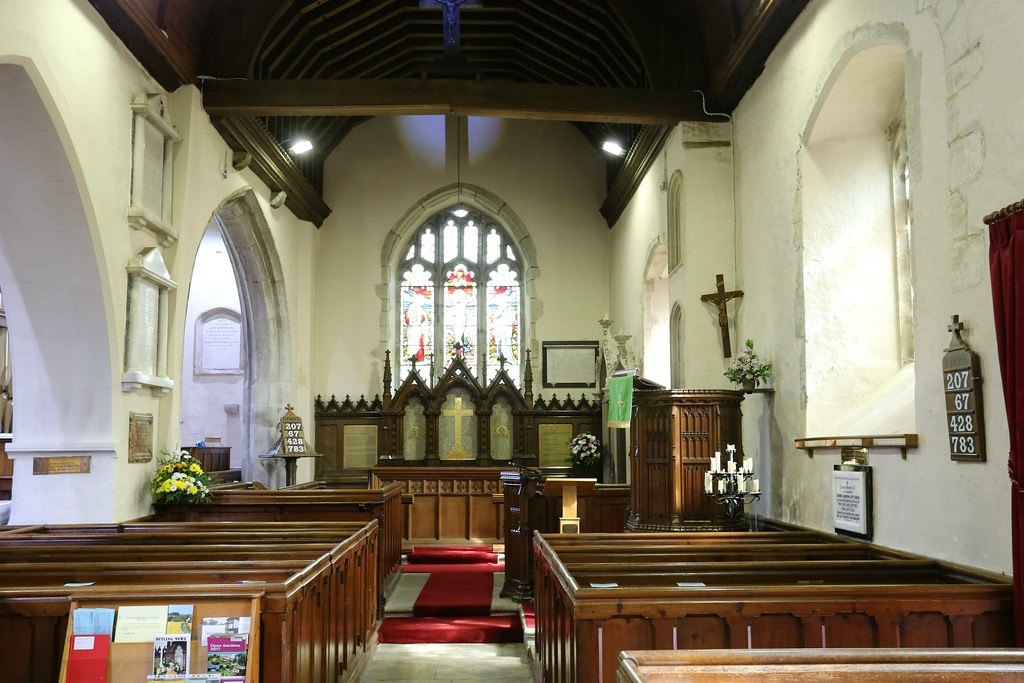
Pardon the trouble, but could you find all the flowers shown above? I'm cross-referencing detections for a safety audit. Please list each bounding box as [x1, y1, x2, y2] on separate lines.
[722, 339, 773, 385]
[149, 445, 216, 503]
[562, 432, 600, 466]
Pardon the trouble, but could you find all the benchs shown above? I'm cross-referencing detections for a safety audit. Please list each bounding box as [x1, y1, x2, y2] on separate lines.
[0, 519, 380, 683]
[533, 529, 1024, 683]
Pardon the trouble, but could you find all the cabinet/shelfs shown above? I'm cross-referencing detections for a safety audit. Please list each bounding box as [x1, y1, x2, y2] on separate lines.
[181, 447, 232, 473]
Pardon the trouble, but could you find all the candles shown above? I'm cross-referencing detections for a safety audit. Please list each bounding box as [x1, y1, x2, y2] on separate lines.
[703, 444, 759, 495]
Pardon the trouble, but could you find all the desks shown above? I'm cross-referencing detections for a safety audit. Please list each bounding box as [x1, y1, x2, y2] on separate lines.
[614, 648, 1024, 683]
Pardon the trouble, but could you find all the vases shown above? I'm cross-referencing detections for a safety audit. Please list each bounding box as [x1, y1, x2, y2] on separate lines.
[151, 499, 188, 522]
[743, 379, 755, 389]
[573, 457, 601, 483]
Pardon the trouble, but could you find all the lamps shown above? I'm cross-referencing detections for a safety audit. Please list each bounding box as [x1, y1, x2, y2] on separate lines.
[280, 138, 314, 157]
[602, 137, 630, 159]
